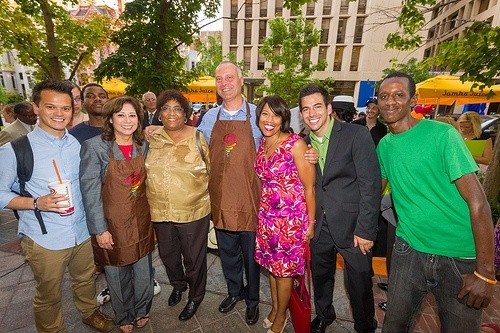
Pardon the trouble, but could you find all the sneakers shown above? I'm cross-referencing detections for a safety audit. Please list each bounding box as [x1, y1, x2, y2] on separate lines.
[96, 287, 111, 304]
[153, 278, 161, 296]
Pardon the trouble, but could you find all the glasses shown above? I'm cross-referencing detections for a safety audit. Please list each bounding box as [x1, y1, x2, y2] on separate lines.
[73, 96, 82, 103]
[161, 106, 185, 113]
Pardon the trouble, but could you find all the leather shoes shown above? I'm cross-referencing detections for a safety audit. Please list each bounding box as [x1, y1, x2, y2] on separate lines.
[378, 302, 387, 311]
[179, 300, 200, 320]
[311, 315, 334, 333]
[245, 301, 259, 325]
[168, 284, 187, 306]
[378, 282, 388, 291]
[218, 290, 246, 312]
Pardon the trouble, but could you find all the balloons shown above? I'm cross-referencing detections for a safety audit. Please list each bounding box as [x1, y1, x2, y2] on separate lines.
[411, 105, 431, 118]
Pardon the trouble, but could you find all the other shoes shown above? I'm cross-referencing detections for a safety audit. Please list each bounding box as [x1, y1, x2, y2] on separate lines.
[263, 318, 272, 329]
[83, 310, 114, 332]
[266, 314, 288, 333]
[94, 262, 104, 281]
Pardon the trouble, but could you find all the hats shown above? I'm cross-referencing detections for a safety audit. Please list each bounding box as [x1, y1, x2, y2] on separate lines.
[365, 97, 378, 106]
[331, 95, 353, 111]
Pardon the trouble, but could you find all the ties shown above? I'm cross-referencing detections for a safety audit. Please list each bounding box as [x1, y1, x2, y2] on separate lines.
[149, 114, 153, 124]
[31, 125, 34, 131]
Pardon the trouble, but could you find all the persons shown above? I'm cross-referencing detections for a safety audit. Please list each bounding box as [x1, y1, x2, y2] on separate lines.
[144, 60, 320, 323]
[430, 107, 493, 176]
[0, 102, 37, 147]
[254, 96, 316, 333]
[378, 71, 497, 333]
[353, 97, 388, 148]
[68, 85, 109, 143]
[0, 81, 115, 333]
[186, 103, 213, 127]
[79, 96, 150, 333]
[66, 84, 90, 130]
[299, 84, 383, 333]
[143, 93, 210, 320]
[332, 95, 356, 123]
[142, 91, 163, 130]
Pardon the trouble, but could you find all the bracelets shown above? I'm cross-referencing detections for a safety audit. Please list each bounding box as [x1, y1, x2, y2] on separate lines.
[474, 270, 497, 285]
[34, 197, 40, 211]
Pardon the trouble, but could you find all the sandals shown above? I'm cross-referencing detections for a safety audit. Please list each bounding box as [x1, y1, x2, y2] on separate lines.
[120, 322, 134, 333]
[136, 312, 149, 327]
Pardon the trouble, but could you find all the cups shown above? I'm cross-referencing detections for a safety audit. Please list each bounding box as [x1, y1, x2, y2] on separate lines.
[48, 180, 75, 216]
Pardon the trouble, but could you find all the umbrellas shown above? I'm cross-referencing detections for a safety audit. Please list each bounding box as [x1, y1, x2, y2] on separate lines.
[90, 73, 500, 119]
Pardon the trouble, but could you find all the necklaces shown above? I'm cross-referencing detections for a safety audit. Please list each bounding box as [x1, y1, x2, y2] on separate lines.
[266, 131, 282, 154]
